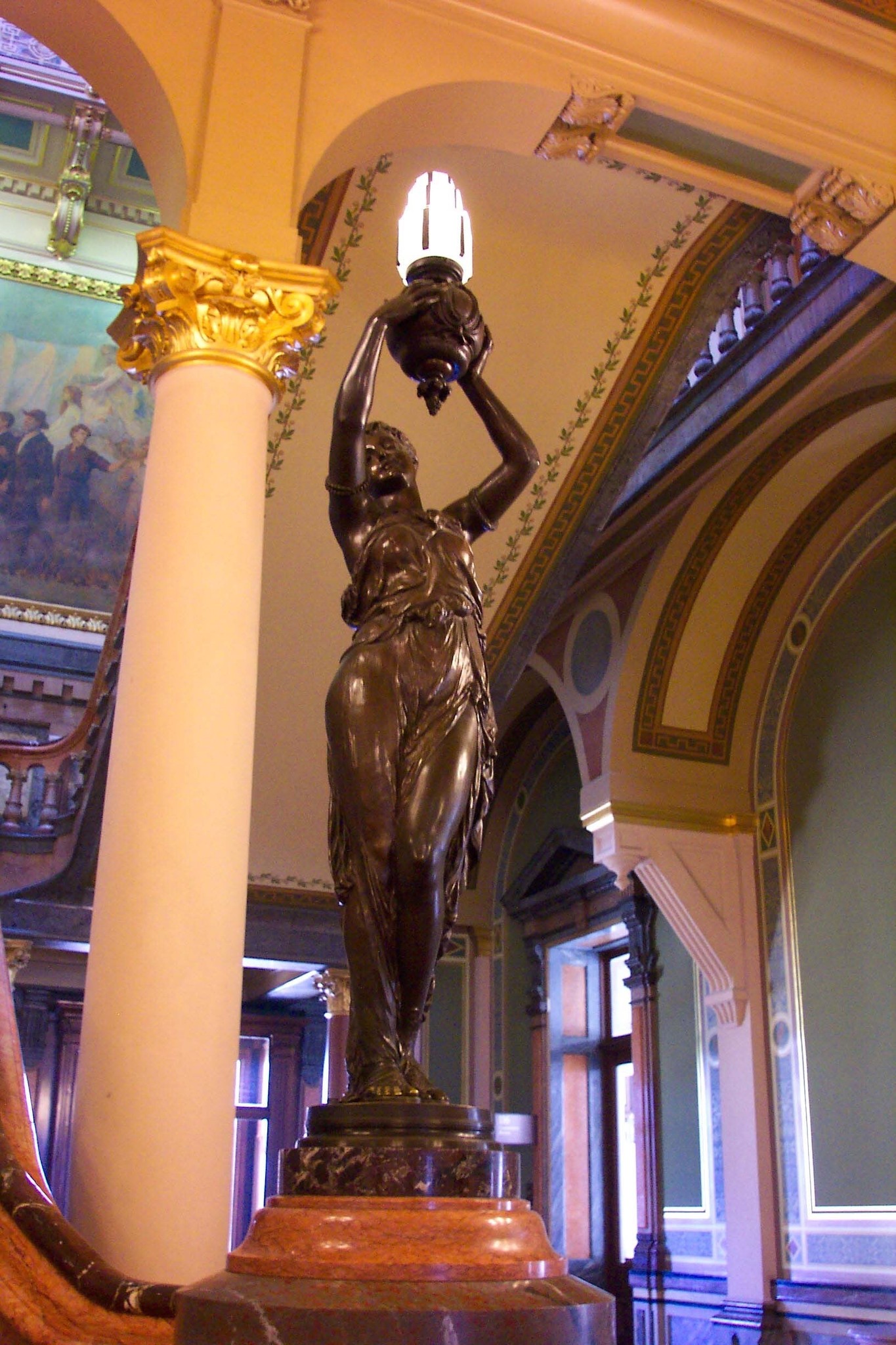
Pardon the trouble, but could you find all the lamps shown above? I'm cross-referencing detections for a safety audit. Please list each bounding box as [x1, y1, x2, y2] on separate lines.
[385, 170, 485, 415]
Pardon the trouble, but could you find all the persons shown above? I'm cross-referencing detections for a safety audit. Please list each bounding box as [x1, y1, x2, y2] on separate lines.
[318, 278, 540, 1097]
